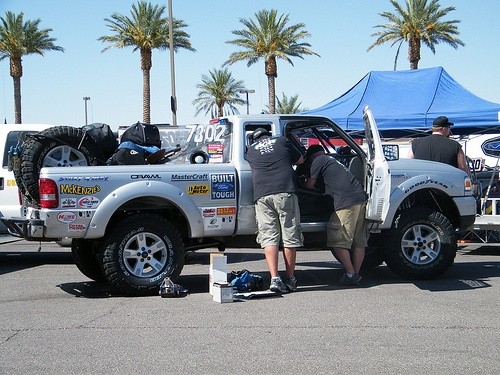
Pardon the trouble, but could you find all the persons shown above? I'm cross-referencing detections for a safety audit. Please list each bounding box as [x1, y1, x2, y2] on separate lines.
[300, 145, 371, 285]
[408, 116, 470, 174]
[245, 128, 304, 294]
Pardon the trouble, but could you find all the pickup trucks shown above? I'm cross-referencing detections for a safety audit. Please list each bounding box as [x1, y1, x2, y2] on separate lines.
[1, 104, 477, 295]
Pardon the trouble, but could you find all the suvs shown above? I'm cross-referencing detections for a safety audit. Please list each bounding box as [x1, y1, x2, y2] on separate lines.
[0, 124, 55, 218]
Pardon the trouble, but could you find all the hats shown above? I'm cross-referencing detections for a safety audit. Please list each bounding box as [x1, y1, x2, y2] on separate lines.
[252, 128, 272, 140]
[432, 116, 454, 126]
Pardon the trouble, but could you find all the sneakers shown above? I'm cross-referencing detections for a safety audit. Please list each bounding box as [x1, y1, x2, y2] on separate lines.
[269, 279, 290, 294]
[338, 272, 362, 285]
[282, 275, 298, 292]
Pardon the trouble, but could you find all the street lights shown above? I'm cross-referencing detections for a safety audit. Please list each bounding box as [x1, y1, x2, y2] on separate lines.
[240, 90, 255, 115]
[83, 97, 90, 125]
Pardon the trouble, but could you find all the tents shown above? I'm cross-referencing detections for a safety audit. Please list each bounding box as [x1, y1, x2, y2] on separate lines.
[299, 66, 500, 216]
